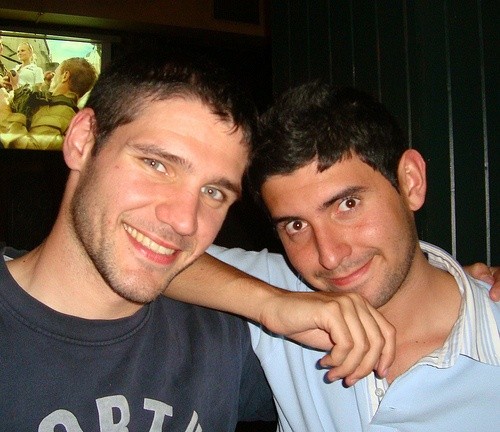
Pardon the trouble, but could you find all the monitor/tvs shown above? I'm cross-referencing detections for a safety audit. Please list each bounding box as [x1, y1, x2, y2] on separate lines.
[0, 30, 111, 149]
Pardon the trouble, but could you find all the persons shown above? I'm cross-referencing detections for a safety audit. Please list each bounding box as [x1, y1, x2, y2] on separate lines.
[161, 79, 499, 432]
[0, 36, 96, 150]
[0, 54, 500, 432]
